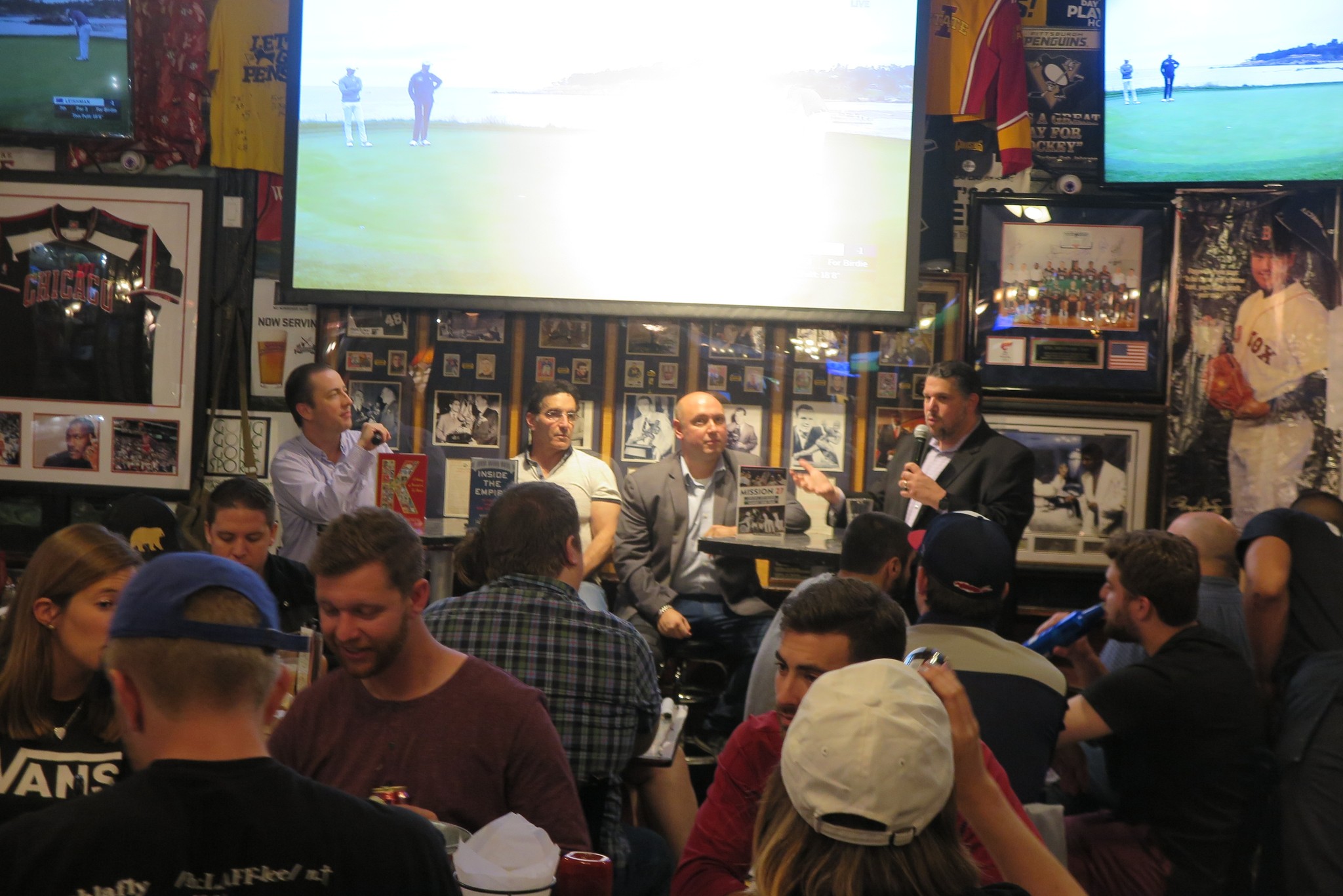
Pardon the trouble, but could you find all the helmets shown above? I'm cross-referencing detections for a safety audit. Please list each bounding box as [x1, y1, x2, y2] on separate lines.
[1240, 209, 1300, 253]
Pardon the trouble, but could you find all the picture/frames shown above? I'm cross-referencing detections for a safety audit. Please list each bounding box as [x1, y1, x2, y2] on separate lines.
[983, 394, 1174, 574]
[958, 189, 1174, 403]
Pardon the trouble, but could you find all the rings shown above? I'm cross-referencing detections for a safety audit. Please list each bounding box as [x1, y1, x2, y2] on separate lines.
[85, 455, 90, 461]
[87, 450, 93, 456]
[88, 445, 94, 451]
[90, 438, 96, 443]
[904, 480, 907, 489]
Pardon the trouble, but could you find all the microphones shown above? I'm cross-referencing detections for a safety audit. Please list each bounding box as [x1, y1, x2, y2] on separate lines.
[366, 418, 382, 444]
[908, 424, 930, 472]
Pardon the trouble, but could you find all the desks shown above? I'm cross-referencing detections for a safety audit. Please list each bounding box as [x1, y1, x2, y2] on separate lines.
[410, 516, 462, 605]
[699, 526, 850, 566]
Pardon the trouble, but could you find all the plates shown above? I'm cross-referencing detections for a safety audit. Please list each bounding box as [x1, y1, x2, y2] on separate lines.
[429, 819, 471, 875]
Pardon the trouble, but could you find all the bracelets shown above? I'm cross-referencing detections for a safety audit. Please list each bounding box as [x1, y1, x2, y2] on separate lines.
[655, 604, 673, 622]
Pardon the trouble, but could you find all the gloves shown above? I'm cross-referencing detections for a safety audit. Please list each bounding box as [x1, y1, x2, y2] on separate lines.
[1201, 352, 1266, 422]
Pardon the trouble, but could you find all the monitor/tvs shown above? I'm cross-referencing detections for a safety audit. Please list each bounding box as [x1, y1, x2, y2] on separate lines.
[283, 0, 930, 326]
[0, 1, 136, 146]
[1099, 0, 1343, 193]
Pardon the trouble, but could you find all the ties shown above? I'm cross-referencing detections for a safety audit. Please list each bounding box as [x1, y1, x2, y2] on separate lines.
[642, 417, 647, 437]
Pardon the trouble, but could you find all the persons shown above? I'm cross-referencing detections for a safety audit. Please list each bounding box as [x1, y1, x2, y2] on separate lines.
[332, 1, 1343, 526]
[201, 481, 344, 679]
[790, 359, 1035, 625]
[1234, 491, 1343, 895]
[741, 659, 1091, 896]
[1031, 509, 1268, 895]
[666, 579, 1043, 896]
[744, 512, 914, 722]
[43, 418, 98, 470]
[420, 481, 699, 896]
[510, 379, 621, 612]
[612, 392, 811, 759]
[271, 363, 395, 571]
[0, 491, 197, 808]
[905, 510, 1070, 803]
[265, 505, 593, 856]
[114, 418, 176, 473]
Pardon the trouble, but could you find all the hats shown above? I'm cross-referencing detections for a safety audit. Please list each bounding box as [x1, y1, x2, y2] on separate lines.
[106, 551, 310, 652]
[780, 657, 955, 847]
[912, 509, 1015, 595]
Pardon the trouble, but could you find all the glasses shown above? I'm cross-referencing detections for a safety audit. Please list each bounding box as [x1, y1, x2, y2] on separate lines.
[538, 409, 584, 424]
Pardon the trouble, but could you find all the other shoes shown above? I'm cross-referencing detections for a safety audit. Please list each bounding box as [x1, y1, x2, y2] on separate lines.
[695, 720, 730, 756]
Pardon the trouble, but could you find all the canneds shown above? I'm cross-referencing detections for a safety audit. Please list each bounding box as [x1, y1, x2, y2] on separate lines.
[903, 646, 946, 672]
[369, 785, 409, 807]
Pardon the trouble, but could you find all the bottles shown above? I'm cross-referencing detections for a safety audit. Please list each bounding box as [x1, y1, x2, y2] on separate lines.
[559, 851, 613, 896]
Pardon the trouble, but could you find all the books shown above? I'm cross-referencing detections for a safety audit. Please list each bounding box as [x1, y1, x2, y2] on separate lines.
[377, 450, 428, 532]
[468, 456, 517, 526]
[738, 464, 788, 540]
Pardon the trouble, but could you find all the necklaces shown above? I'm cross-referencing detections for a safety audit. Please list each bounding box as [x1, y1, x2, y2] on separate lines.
[1, 553, 463, 895]
[46, 703, 82, 739]
